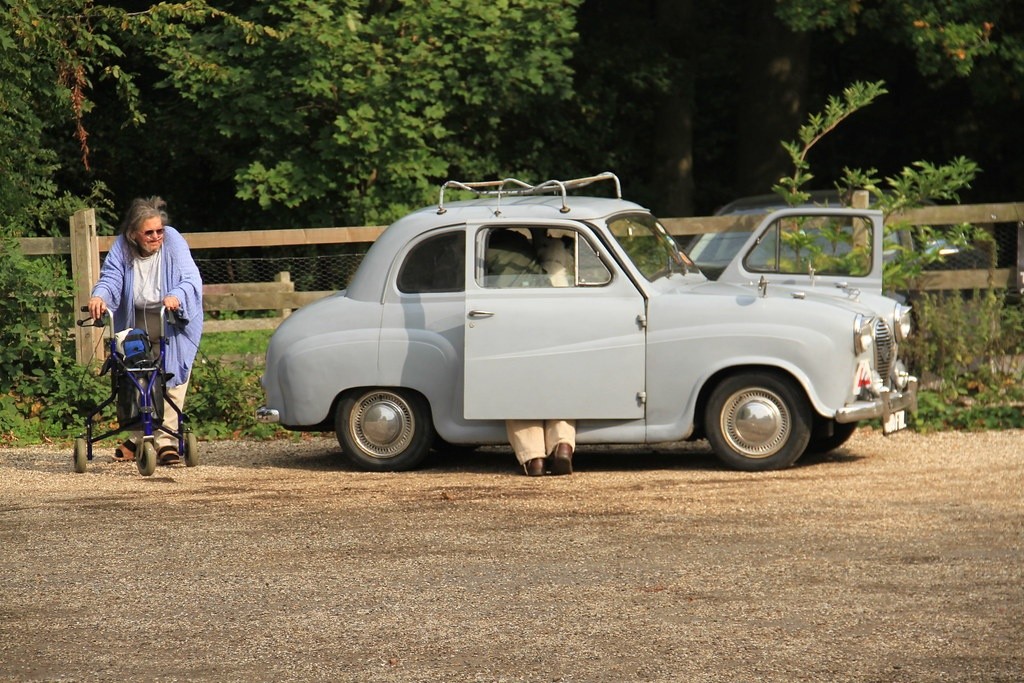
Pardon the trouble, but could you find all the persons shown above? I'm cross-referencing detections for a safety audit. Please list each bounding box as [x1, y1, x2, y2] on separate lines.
[87, 196, 204, 464]
[484, 226, 576, 479]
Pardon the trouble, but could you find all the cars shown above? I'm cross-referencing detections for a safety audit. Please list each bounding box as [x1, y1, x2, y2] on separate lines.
[254, 172, 917, 472]
[684, 187, 979, 281]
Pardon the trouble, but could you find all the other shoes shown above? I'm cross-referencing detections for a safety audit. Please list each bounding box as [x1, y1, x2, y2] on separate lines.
[525, 459, 547, 476]
[552, 443, 573, 474]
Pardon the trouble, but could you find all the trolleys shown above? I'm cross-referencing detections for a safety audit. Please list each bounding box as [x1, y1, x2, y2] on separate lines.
[73, 304, 198, 475]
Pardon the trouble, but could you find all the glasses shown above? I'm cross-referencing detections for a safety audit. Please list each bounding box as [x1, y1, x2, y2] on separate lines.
[134, 228, 166, 237]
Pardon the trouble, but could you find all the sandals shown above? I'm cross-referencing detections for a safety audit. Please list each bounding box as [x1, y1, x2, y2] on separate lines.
[159, 451, 180, 464]
[111, 445, 137, 461]
